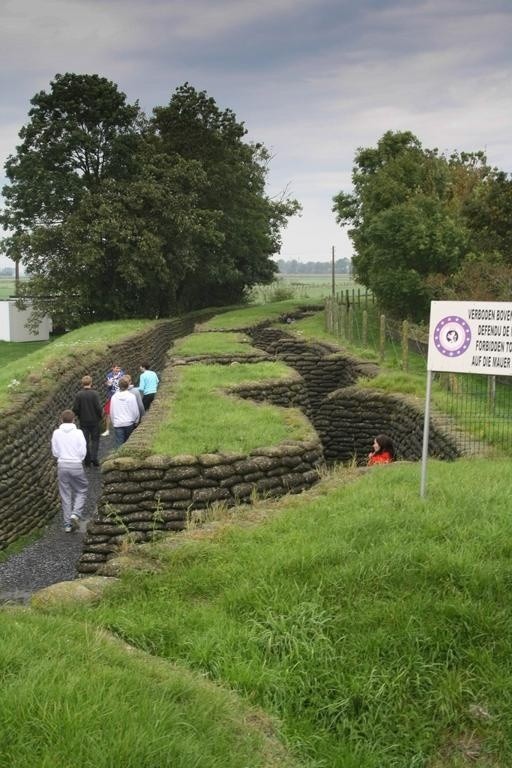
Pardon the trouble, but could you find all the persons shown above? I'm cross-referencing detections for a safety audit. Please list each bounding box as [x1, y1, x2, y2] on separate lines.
[100, 363, 125, 437]
[51, 410, 89, 533]
[123, 374, 145, 419]
[366, 434, 395, 467]
[136, 362, 160, 412]
[109, 378, 140, 449]
[72, 376, 105, 467]
[286, 317, 292, 324]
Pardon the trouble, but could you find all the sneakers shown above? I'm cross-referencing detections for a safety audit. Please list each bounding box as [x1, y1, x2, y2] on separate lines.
[84, 456, 99, 467]
[65, 514, 80, 532]
[100, 429, 109, 437]
